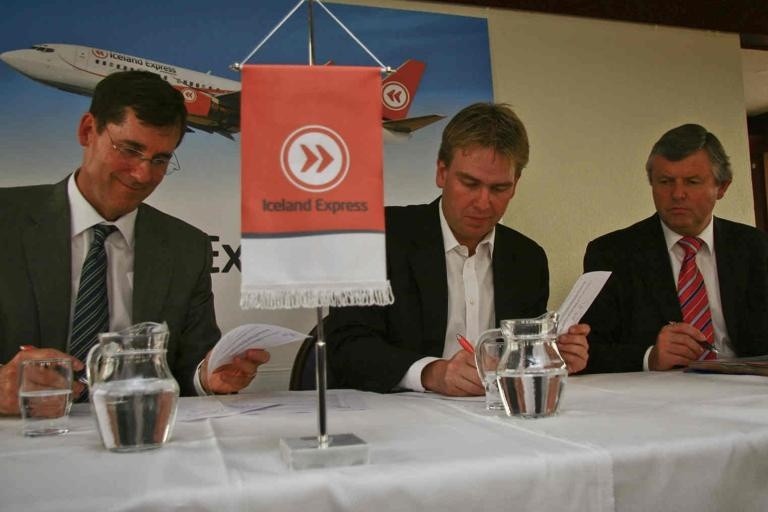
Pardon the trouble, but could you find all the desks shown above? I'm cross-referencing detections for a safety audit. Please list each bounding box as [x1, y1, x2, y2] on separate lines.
[0, 371, 768, 512]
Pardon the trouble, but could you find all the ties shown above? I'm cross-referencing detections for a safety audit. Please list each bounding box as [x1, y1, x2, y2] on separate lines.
[69, 225, 118, 404]
[677, 237, 717, 361]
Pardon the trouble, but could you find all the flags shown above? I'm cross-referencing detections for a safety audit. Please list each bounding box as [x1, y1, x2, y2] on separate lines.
[239, 63, 394, 309]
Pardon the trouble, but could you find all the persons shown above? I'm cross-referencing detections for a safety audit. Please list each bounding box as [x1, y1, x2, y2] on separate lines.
[327, 101, 592, 397]
[0, 71, 270, 417]
[566, 124, 768, 376]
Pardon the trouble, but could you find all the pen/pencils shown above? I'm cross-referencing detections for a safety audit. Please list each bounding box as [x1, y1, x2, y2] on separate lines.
[455, 333, 474, 355]
[16, 340, 88, 386]
[668, 319, 720, 355]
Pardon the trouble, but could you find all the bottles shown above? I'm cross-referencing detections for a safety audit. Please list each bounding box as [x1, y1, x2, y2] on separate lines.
[472, 313, 570, 419]
[84, 320, 177, 457]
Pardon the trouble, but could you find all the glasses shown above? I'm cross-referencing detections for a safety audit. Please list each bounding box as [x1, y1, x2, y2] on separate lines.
[105, 126, 181, 175]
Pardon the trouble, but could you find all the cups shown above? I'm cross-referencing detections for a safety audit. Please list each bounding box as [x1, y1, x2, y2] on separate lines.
[479, 340, 507, 412]
[18, 355, 73, 441]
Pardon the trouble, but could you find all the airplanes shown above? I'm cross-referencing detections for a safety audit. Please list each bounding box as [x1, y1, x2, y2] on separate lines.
[0, 43, 447, 142]
